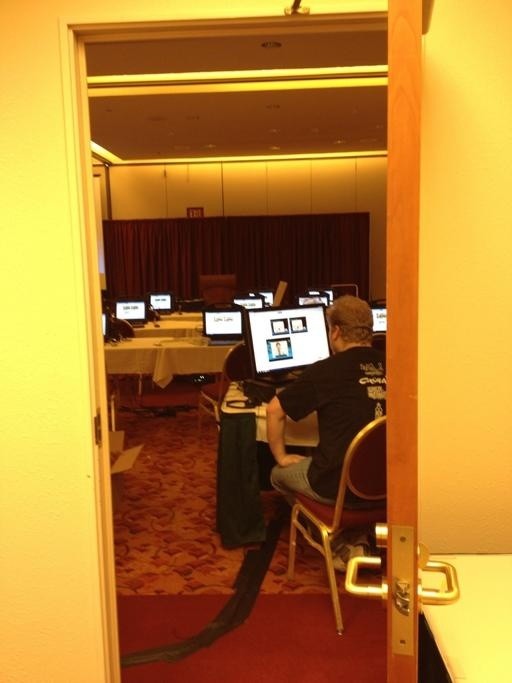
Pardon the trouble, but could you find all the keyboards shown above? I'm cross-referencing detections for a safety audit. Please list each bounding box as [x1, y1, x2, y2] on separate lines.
[208, 340, 236, 346]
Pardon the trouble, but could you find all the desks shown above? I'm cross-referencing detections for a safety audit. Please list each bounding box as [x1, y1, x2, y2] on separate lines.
[104, 311, 320, 447]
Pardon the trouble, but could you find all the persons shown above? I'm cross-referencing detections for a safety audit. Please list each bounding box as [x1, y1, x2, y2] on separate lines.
[275, 343, 287, 358]
[267, 295, 386, 576]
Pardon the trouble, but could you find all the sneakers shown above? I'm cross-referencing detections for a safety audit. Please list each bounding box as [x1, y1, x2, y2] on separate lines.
[333, 543, 370, 573]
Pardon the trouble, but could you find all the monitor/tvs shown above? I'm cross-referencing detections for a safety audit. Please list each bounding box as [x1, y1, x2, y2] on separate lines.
[116, 300, 147, 323]
[244, 304, 332, 383]
[203, 308, 245, 340]
[297, 295, 329, 308]
[248, 292, 274, 308]
[101, 310, 110, 342]
[149, 292, 174, 311]
[308, 289, 334, 302]
[370, 305, 386, 332]
[232, 295, 265, 309]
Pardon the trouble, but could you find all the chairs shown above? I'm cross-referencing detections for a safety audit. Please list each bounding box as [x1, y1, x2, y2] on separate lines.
[287, 416, 386, 635]
